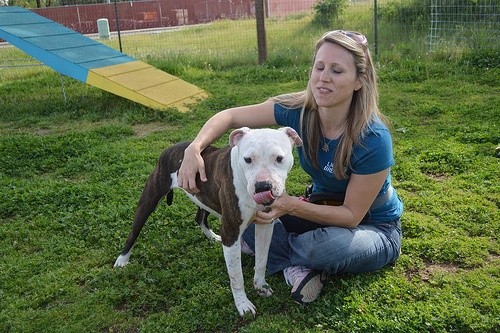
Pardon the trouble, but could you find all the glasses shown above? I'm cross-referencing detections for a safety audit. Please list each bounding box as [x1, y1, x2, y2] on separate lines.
[334, 30, 368, 72]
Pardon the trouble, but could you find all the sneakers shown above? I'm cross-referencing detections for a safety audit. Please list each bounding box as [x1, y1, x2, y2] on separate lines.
[284, 267, 327, 304]
[240, 238, 256, 256]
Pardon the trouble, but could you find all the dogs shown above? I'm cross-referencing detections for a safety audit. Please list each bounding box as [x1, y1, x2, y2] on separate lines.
[109, 123, 304, 318]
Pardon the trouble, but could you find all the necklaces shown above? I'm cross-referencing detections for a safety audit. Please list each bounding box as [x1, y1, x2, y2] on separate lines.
[322, 116, 349, 153]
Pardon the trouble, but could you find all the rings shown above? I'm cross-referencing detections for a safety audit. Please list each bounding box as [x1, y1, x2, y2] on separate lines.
[271, 217, 274, 224]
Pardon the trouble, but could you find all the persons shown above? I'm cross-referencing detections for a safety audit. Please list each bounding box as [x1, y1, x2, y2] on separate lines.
[176, 29, 404, 303]
